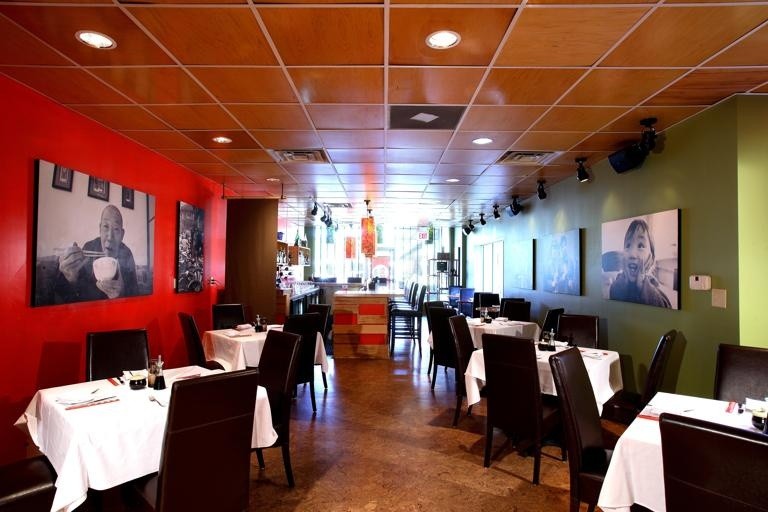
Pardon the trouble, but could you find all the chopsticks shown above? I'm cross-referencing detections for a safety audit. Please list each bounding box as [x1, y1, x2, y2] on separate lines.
[50, 246, 107, 257]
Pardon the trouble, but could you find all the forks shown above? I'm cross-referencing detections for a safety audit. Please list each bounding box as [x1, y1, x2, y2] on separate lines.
[147, 397, 166, 409]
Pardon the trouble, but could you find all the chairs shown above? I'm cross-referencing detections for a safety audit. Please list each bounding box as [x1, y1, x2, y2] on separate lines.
[79, 327, 157, 383]
[546, 347, 615, 512]
[386, 281, 427, 359]
[176, 302, 333, 490]
[153, 371, 264, 512]
[657, 411, 767, 512]
[425, 282, 625, 485]
[599, 329, 675, 425]
[713, 342, 767, 402]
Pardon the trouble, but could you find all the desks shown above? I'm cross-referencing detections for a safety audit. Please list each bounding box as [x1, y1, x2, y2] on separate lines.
[463, 336, 622, 465]
[462, 315, 541, 351]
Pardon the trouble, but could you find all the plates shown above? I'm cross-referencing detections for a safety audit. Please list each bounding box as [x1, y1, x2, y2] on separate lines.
[54, 393, 95, 404]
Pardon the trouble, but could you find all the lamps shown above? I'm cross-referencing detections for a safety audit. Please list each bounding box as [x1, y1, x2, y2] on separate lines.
[573, 154, 590, 184]
[605, 116, 657, 175]
[535, 177, 547, 200]
[462, 203, 500, 234]
[308, 201, 333, 228]
[344, 199, 375, 262]
[507, 193, 522, 215]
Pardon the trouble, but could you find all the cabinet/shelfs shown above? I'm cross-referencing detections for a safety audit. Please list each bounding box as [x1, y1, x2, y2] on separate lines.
[275, 239, 311, 267]
[290, 291, 319, 315]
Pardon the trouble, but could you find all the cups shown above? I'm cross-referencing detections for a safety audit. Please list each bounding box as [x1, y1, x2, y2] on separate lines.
[478, 303, 501, 323]
[751, 407, 765, 431]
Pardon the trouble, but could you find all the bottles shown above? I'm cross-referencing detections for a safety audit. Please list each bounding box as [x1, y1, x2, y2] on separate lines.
[276, 229, 316, 297]
[128, 353, 167, 391]
[251, 313, 269, 334]
[538, 327, 556, 351]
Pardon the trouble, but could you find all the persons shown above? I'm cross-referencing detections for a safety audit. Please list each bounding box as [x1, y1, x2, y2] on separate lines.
[610, 219, 672, 308]
[55, 205, 138, 303]
[543, 239, 560, 291]
[556, 234, 575, 292]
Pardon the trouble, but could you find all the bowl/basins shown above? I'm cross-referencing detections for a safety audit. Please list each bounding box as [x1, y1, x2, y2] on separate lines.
[90, 257, 120, 281]
